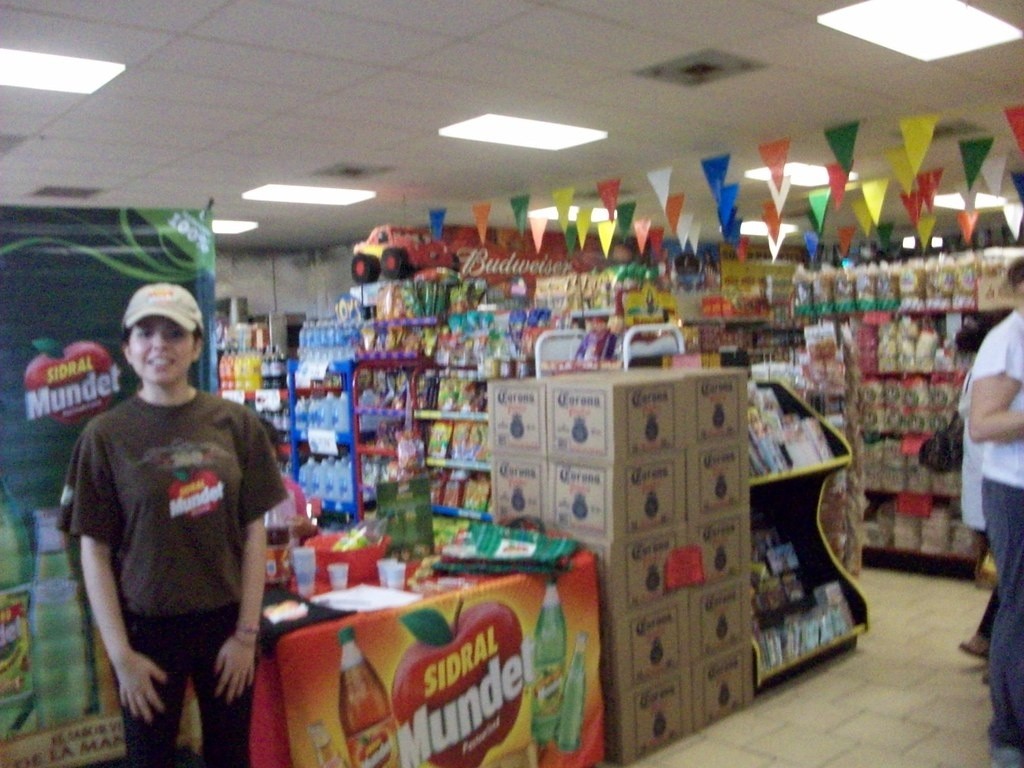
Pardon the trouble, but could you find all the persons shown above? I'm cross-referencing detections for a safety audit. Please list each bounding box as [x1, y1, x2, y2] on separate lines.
[261, 419, 320, 544]
[958, 256, 1024, 768]
[57, 284, 288, 768]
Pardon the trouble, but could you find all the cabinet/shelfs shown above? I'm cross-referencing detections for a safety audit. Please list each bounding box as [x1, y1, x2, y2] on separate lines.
[207, 227, 1018, 687]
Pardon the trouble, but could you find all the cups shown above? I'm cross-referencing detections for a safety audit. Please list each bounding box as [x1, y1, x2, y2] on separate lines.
[291, 547, 315, 598]
[327, 563, 348, 588]
[377, 557, 407, 591]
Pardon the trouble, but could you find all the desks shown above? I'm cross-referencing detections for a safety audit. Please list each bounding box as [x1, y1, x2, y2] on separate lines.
[242, 536, 602, 768]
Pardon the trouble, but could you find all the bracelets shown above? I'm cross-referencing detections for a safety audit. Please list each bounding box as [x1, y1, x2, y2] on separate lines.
[235, 623, 259, 633]
[233, 635, 255, 647]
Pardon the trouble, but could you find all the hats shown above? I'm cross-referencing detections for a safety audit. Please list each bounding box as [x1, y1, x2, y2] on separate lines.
[121, 284, 204, 339]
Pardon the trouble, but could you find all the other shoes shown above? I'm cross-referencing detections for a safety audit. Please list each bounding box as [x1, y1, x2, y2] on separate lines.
[990, 742, 1022, 768]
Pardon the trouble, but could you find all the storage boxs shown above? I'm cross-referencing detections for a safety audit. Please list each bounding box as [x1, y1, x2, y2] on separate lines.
[481, 367, 753, 765]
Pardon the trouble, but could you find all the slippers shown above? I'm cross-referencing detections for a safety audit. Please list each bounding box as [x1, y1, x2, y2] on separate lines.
[958, 638, 991, 658]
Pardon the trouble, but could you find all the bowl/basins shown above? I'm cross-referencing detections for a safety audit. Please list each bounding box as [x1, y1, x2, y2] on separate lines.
[302, 532, 390, 579]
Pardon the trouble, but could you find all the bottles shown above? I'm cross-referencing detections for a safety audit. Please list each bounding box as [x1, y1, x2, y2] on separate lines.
[0, 478, 95, 742]
[216, 318, 362, 504]
[261, 501, 292, 591]
[530, 576, 589, 751]
[337, 628, 401, 768]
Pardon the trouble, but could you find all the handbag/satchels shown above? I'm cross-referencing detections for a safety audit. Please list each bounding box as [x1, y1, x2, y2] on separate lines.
[918, 409, 966, 471]
